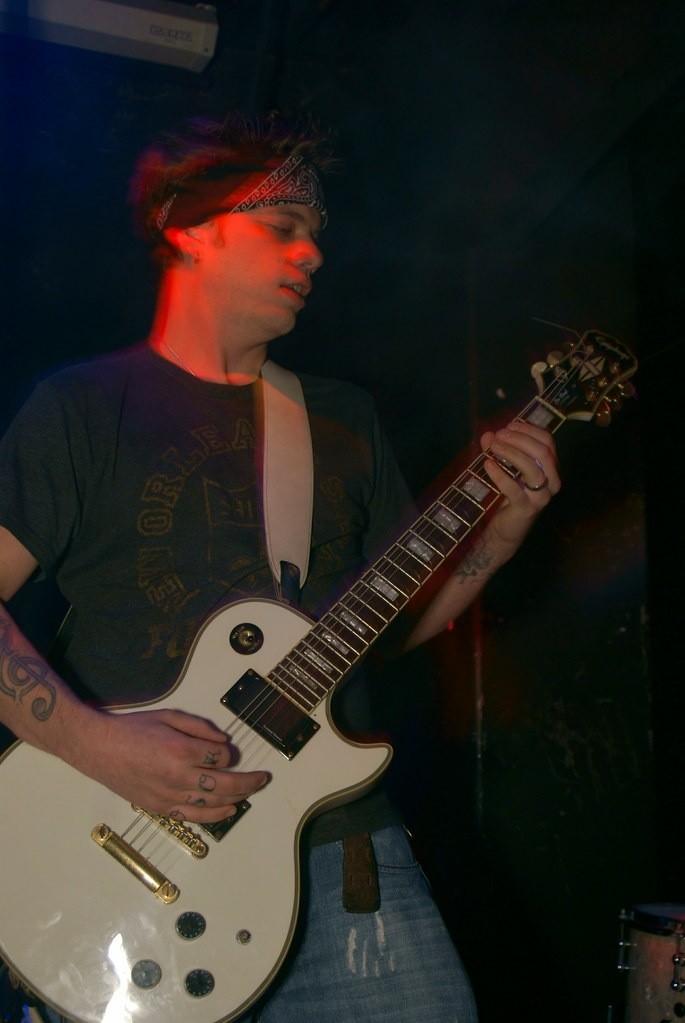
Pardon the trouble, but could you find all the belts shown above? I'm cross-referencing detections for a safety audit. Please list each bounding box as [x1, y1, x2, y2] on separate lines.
[343, 833, 380, 914]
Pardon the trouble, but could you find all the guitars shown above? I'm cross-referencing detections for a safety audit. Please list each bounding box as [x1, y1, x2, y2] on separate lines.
[1, 325, 648, 1023]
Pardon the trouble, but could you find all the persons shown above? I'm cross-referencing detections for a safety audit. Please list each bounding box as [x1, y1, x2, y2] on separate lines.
[0, 109, 561, 1023]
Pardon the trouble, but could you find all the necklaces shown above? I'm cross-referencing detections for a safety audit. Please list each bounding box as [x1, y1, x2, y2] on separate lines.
[151, 330, 195, 375]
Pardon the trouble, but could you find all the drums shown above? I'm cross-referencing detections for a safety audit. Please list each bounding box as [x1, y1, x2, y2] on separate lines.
[614, 897, 685, 1023]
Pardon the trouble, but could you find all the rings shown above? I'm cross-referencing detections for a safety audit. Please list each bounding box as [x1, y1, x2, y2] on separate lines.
[525, 477, 548, 491]
